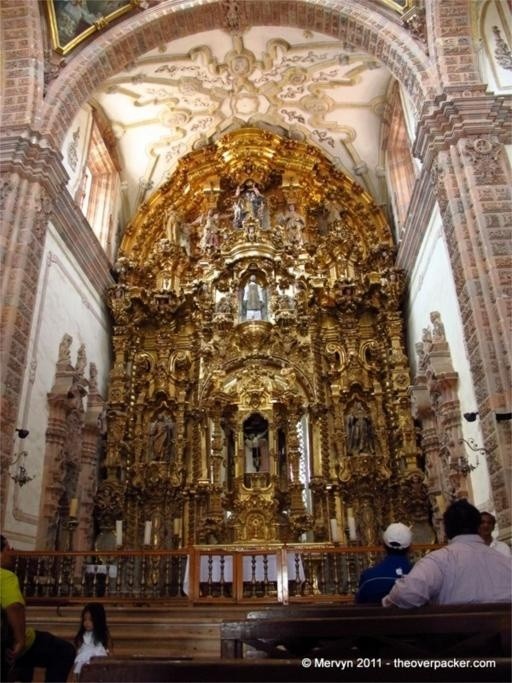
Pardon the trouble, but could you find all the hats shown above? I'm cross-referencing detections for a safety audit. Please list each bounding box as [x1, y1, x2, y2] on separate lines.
[381, 521, 414, 550]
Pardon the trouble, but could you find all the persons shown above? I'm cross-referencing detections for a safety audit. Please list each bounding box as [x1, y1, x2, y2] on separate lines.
[243, 428, 268, 473]
[381, 501, 512, 610]
[442, 473, 469, 504]
[72, 600, 115, 675]
[148, 413, 174, 462]
[400, 474, 430, 511]
[232, 198, 244, 229]
[0, 534, 27, 682]
[477, 509, 512, 558]
[414, 342, 425, 369]
[278, 203, 308, 247]
[18, 625, 77, 682]
[89, 361, 99, 383]
[252, 197, 267, 226]
[355, 522, 414, 603]
[75, 344, 87, 372]
[430, 311, 446, 344]
[57, 333, 74, 365]
[248, 181, 265, 199]
[358, 409, 377, 454]
[192, 207, 229, 250]
[421, 328, 431, 353]
[231, 185, 245, 198]
[344, 408, 361, 455]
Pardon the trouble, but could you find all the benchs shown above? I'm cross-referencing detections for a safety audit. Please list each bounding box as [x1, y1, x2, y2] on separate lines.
[79, 601, 511, 681]
